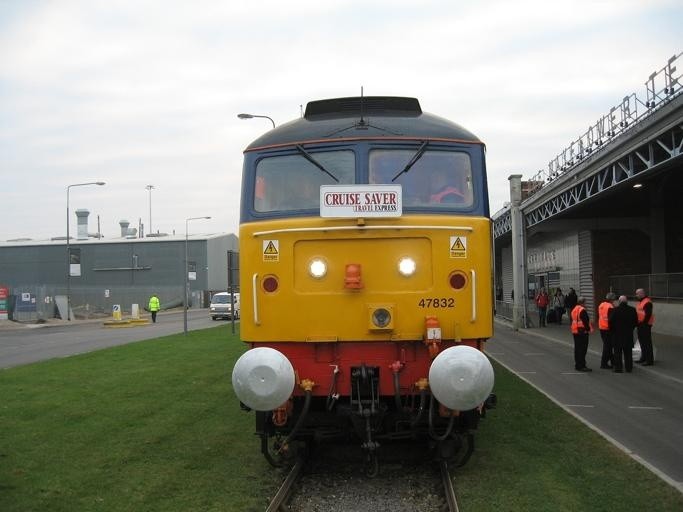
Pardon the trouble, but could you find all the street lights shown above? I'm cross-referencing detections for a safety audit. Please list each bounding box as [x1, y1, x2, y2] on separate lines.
[560, 308, 566, 313]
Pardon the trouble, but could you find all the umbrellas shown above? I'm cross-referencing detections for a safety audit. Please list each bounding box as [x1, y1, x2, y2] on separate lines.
[580, 367, 593, 371]
[636, 360, 648, 366]
[601, 365, 612, 369]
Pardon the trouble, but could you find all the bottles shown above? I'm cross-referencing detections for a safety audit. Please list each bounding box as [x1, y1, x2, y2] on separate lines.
[606, 293, 617, 299]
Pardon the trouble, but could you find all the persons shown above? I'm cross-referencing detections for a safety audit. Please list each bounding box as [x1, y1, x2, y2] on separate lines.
[494, 286, 578, 328]
[596, 292, 615, 369]
[609, 295, 637, 373]
[148, 294, 160, 323]
[569, 297, 594, 373]
[428, 166, 466, 206]
[634, 287, 655, 367]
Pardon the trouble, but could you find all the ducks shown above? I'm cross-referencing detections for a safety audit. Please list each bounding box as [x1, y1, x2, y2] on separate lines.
[547, 309, 556, 323]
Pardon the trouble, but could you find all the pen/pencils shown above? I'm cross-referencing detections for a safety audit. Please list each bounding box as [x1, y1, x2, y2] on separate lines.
[209, 292, 239, 322]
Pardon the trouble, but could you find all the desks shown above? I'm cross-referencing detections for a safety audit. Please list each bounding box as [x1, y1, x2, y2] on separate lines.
[182, 215, 210, 333]
[144, 185, 155, 233]
[65, 182, 104, 320]
[236, 109, 278, 136]
